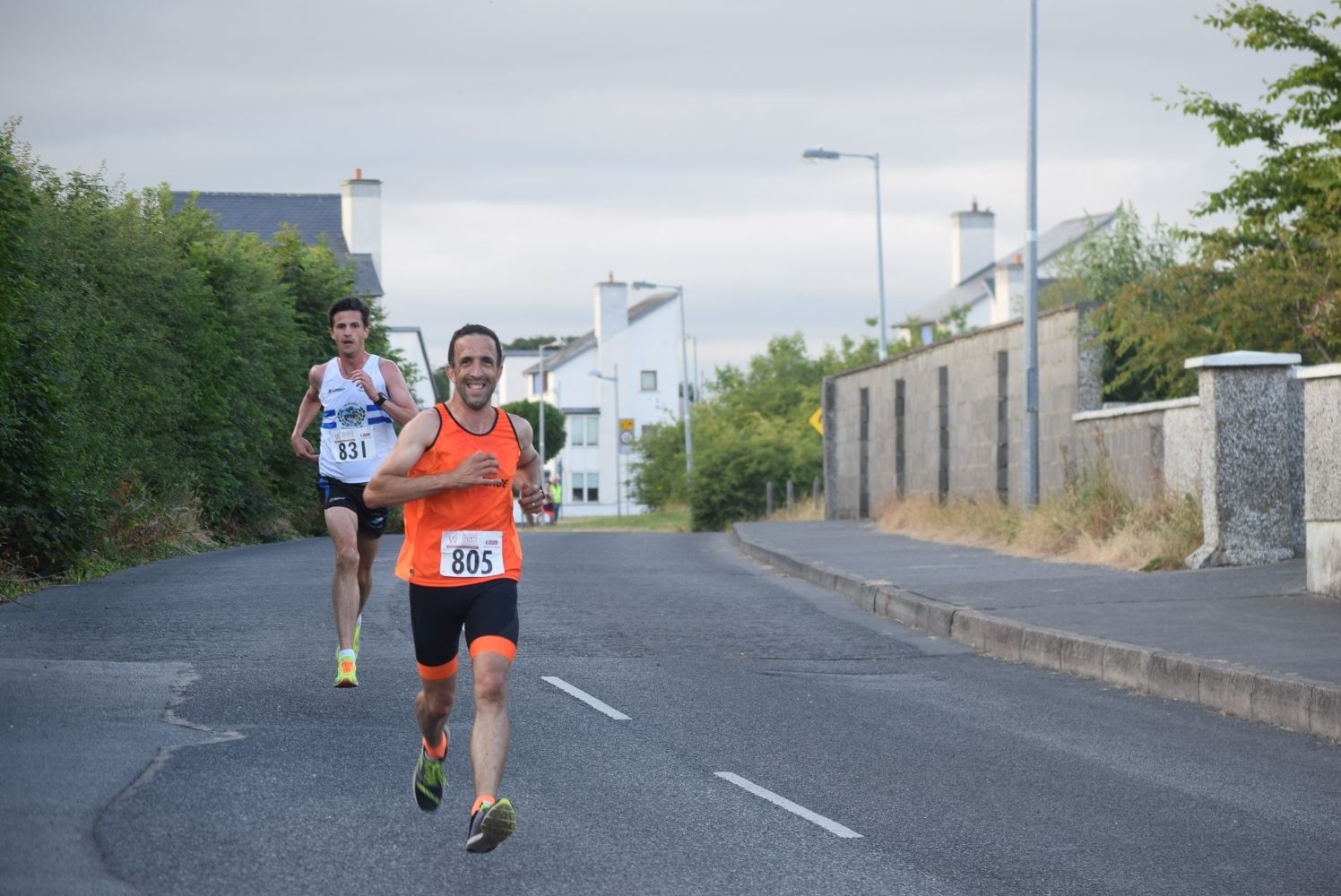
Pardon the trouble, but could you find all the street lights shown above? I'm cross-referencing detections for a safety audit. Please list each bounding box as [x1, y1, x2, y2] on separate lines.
[633, 282, 693, 490]
[538, 341, 565, 492]
[803, 149, 888, 362]
[588, 363, 622, 518]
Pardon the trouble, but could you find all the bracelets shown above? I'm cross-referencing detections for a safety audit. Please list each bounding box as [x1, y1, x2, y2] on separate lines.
[540, 486, 546, 500]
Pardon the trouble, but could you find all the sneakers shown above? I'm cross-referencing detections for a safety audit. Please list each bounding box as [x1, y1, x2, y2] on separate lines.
[465, 798, 515, 853]
[336, 615, 363, 664]
[335, 656, 359, 687]
[412, 725, 450, 814]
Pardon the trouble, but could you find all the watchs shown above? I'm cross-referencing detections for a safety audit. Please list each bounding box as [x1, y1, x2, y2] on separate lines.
[374, 391, 387, 408]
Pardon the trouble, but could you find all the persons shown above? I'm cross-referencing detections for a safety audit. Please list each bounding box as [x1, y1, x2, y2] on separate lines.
[362, 326, 546, 852]
[290, 298, 420, 689]
[548, 476, 562, 521]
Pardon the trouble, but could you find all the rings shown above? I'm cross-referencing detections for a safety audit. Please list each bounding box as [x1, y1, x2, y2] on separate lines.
[531, 501, 535, 508]
